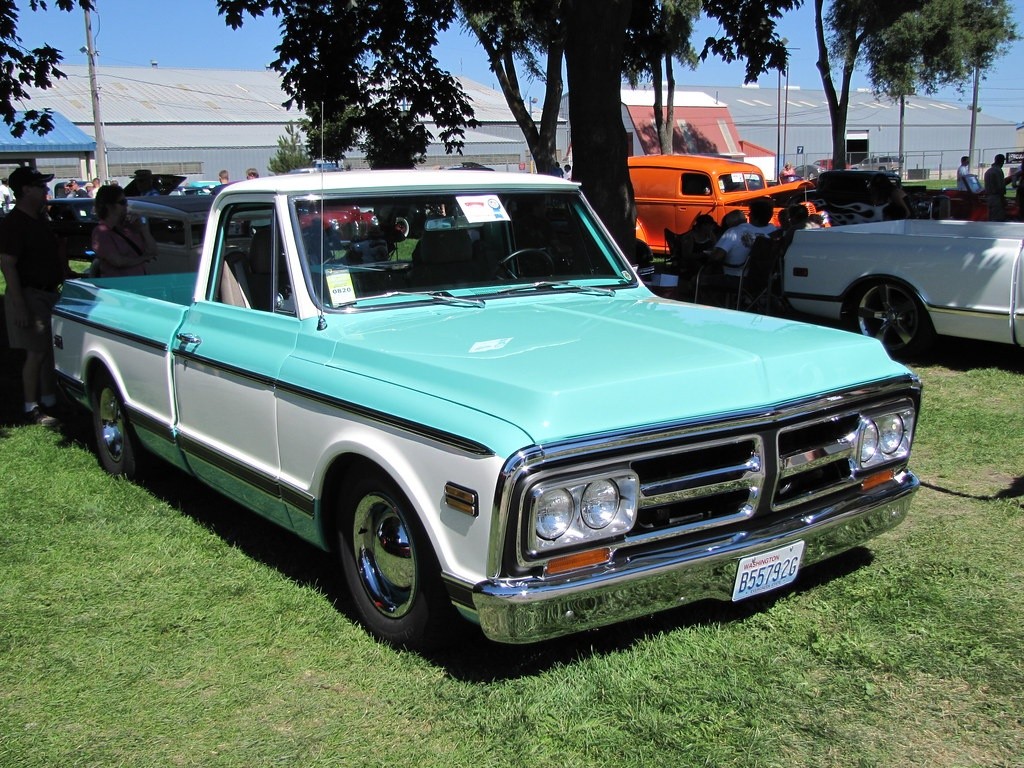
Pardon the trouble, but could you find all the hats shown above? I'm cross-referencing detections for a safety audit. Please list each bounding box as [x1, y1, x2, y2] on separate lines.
[8, 166, 54, 191]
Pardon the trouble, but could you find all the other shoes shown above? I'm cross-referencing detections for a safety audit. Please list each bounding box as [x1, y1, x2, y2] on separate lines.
[23, 404, 57, 428]
[38, 398, 80, 420]
[665, 228, 682, 251]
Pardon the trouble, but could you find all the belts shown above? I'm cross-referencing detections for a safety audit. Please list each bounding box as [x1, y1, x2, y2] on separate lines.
[722, 262, 746, 269]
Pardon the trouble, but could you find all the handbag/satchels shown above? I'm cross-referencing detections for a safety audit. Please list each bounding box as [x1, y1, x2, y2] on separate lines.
[135, 249, 154, 275]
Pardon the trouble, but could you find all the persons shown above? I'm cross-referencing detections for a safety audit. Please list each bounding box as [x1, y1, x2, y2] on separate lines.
[976, 153, 1024, 222]
[678, 198, 824, 308]
[780, 162, 795, 182]
[957, 156, 970, 191]
[245, 168, 259, 180]
[304, 218, 388, 265]
[210, 170, 231, 196]
[0, 166, 158, 426]
[555, 162, 572, 181]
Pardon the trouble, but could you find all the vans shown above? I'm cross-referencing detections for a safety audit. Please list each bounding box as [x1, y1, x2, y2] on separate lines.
[125, 194, 217, 273]
[851, 156, 899, 171]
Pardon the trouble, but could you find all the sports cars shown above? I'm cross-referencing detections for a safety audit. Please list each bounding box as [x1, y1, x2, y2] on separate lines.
[927, 174, 1024, 221]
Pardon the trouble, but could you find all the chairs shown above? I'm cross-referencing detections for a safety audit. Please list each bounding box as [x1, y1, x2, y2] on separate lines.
[406, 214, 504, 289]
[662, 223, 793, 316]
[250, 222, 291, 314]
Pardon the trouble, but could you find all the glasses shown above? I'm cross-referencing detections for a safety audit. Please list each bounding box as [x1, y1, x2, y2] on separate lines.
[26, 182, 47, 189]
[110, 198, 128, 207]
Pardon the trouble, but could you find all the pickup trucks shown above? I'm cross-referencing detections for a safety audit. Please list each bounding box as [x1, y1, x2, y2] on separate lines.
[784, 219, 1024, 360]
[51, 169, 923, 650]
[813, 159, 851, 169]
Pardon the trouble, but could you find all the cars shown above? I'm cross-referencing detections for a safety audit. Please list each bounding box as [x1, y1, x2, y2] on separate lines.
[793, 164, 829, 178]
[46, 198, 100, 239]
[628, 154, 831, 255]
[299, 205, 379, 241]
